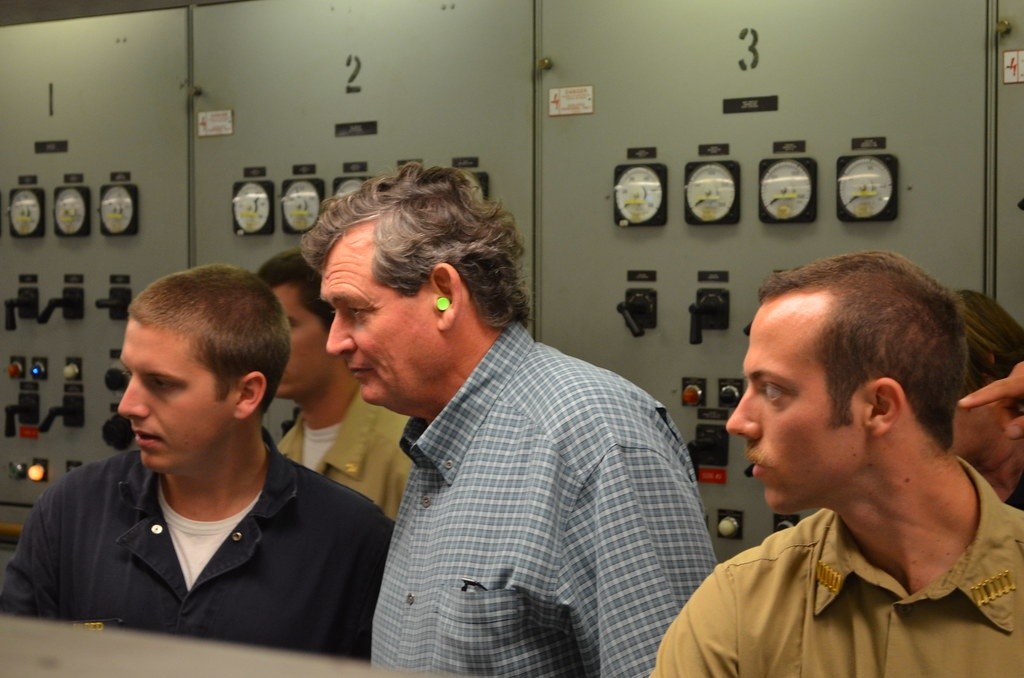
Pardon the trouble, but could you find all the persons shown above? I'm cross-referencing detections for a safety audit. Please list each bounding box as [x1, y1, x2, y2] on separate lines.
[255, 249, 415, 524]
[934, 287, 1024, 518]
[648, 255, 1024, 678]
[958, 360, 1024, 439]
[292, 157, 718, 678]
[2, 262, 395, 664]
